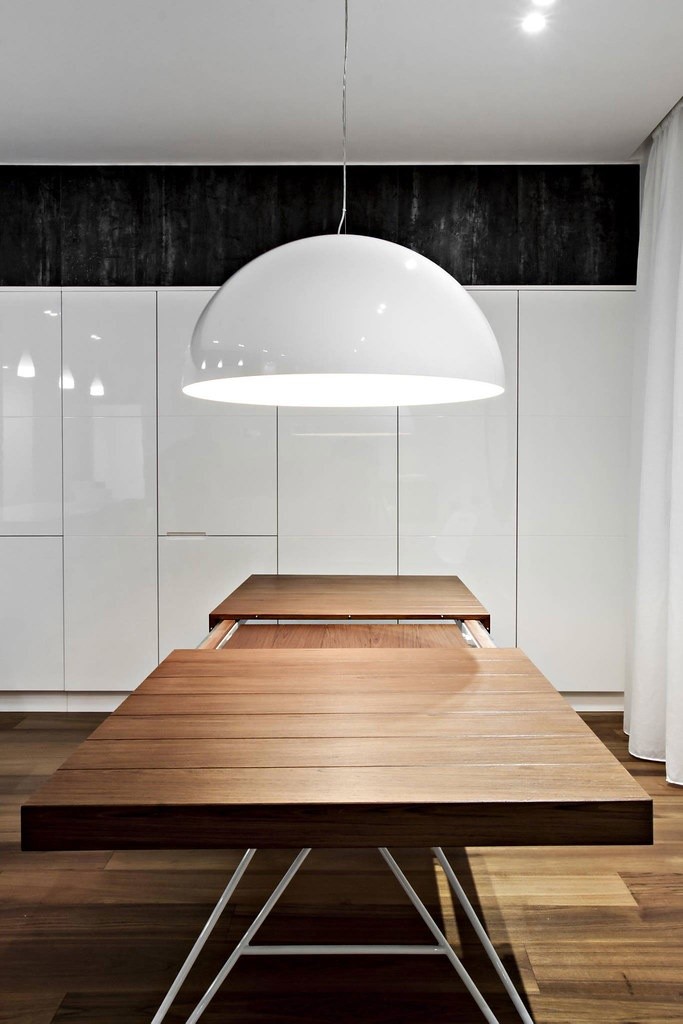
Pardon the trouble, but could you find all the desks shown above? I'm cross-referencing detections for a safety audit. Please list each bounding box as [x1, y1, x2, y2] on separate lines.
[19, 573, 655, 1024]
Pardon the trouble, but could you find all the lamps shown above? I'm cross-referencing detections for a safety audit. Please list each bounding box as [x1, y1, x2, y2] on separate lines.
[180, 0, 509, 408]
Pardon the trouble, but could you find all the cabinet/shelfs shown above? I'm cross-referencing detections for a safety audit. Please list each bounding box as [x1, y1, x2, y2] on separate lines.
[0, 284, 638, 691]
